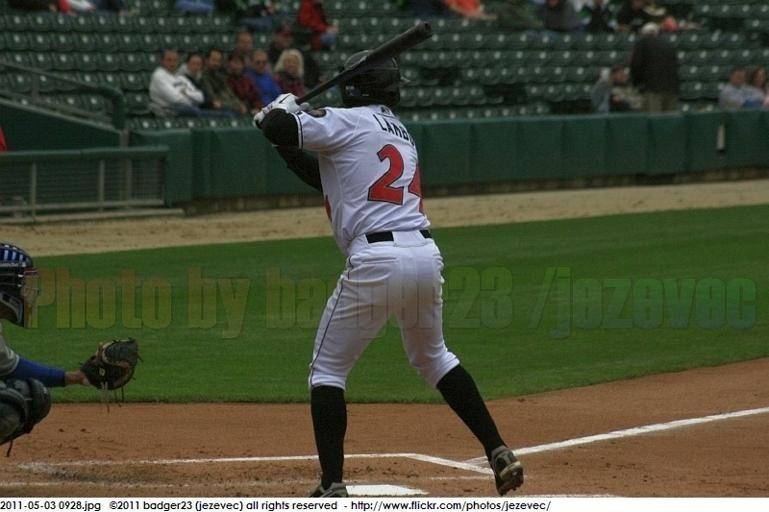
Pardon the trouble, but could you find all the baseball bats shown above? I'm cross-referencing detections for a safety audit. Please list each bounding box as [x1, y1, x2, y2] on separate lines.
[253, 21, 433, 130]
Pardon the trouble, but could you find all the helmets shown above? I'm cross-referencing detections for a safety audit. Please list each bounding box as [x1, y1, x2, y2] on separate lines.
[342, 50, 402, 111]
[0, 243, 38, 320]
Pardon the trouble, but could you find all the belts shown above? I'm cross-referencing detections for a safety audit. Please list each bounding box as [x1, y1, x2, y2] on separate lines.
[366, 229, 433, 243]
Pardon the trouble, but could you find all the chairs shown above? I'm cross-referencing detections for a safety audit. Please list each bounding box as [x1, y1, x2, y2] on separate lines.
[0, 0, 769, 134]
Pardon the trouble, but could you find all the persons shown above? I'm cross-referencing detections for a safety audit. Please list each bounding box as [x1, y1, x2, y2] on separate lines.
[628, 23, 682, 115]
[1, 245, 137, 443]
[442, 2, 682, 38]
[145, 0, 342, 125]
[746, 67, 768, 95]
[717, 66, 768, 108]
[607, 64, 643, 110]
[6, 1, 128, 17]
[253, 47, 525, 497]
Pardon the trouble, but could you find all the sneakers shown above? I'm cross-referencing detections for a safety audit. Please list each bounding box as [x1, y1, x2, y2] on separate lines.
[490, 445, 524, 495]
[309, 481, 349, 497]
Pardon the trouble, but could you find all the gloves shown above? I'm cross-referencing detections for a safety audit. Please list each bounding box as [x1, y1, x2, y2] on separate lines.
[254, 92, 310, 129]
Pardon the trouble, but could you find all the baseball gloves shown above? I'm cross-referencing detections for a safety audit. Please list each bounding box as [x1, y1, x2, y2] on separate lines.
[82, 337, 138, 390]
